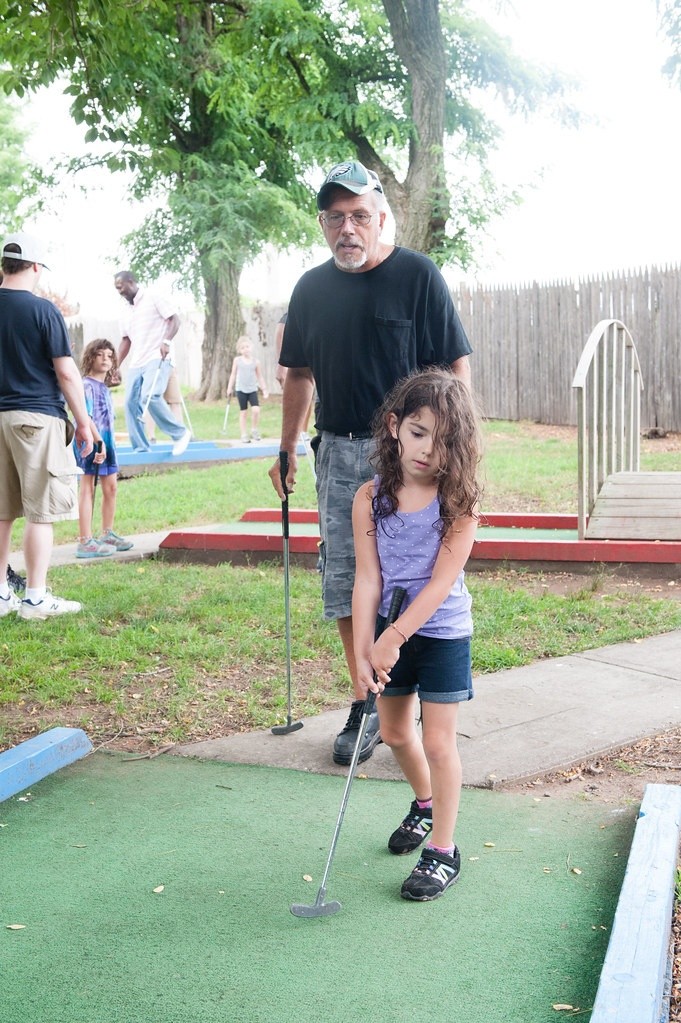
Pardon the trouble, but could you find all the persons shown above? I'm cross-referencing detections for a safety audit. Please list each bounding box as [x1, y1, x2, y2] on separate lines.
[0, 240, 95, 622]
[268, 161, 483, 767]
[0, 272, 317, 595]
[351, 373, 482, 905]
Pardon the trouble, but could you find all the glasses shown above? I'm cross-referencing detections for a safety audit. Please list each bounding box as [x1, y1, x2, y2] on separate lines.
[321, 211, 374, 228]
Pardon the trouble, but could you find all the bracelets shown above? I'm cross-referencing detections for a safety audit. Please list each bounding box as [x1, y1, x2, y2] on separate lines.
[390, 623, 408, 641]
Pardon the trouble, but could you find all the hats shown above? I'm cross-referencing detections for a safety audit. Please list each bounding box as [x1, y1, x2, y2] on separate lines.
[4, 240, 52, 270]
[317, 159, 383, 211]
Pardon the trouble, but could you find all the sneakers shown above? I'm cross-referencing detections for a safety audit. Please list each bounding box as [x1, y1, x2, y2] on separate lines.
[398, 845, 462, 900]
[334, 701, 383, 766]
[388, 801, 433, 855]
[77, 539, 117, 557]
[0, 593, 20, 616]
[18, 594, 82, 620]
[101, 533, 134, 551]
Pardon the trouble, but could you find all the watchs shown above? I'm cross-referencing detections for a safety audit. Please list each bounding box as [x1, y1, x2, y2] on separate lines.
[162, 339, 171, 346]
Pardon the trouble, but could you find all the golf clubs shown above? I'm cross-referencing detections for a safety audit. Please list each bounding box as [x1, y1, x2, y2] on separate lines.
[270, 449, 305, 736]
[136, 357, 164, 425]
[175, 374, 195, 440]
[289, 585, 409, 920]
[91, 440, 102, 519]
[221, 395, 231, 435]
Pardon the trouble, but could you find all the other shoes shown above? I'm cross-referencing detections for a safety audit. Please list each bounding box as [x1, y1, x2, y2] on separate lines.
[6, 568, 26, 593]
[241, 434, 251, 443]
[251, 431, 262, 441]
[299, 433, 311, 441]
[172, 430, 192, 456]
